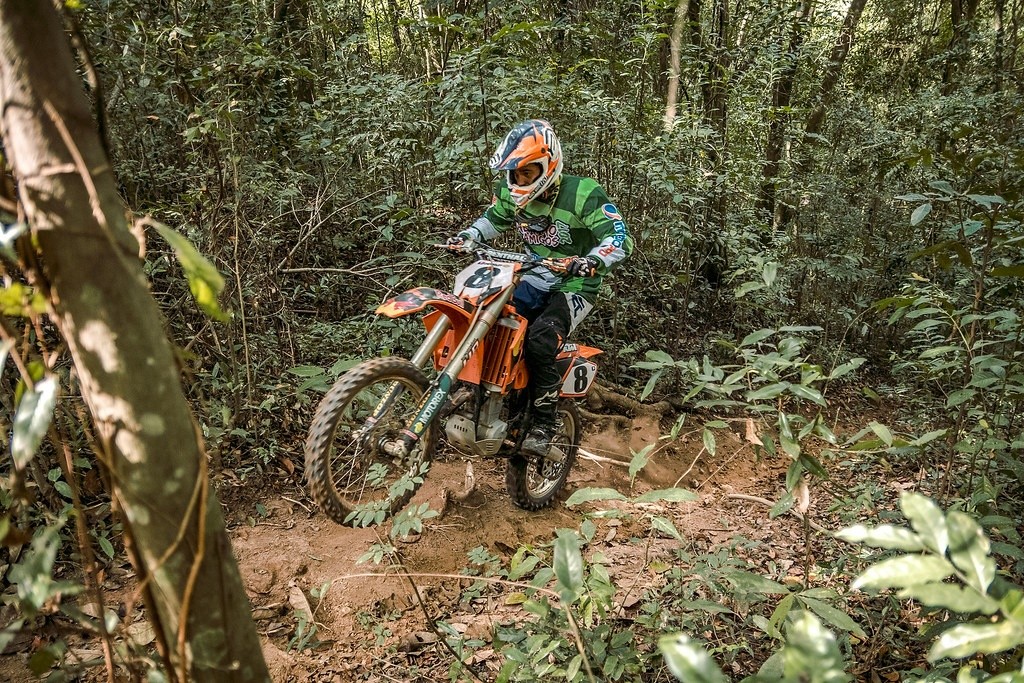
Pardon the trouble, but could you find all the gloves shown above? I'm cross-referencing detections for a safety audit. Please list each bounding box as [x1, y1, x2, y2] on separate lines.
[566, 256, 600, 277]
[446, 236, 468, 258]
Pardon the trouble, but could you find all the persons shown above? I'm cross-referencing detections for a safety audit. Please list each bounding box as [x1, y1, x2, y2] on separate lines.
[447, 119, 633, 456]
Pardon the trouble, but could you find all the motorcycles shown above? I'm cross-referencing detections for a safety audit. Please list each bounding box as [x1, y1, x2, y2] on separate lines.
[303, 241, 609, 528]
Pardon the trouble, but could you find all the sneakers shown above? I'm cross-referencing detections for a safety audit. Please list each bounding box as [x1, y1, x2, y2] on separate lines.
[522, 426, 552, 457]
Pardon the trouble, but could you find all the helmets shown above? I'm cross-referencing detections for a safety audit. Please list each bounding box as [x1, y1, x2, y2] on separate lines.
[489, 119, 564, 208]
